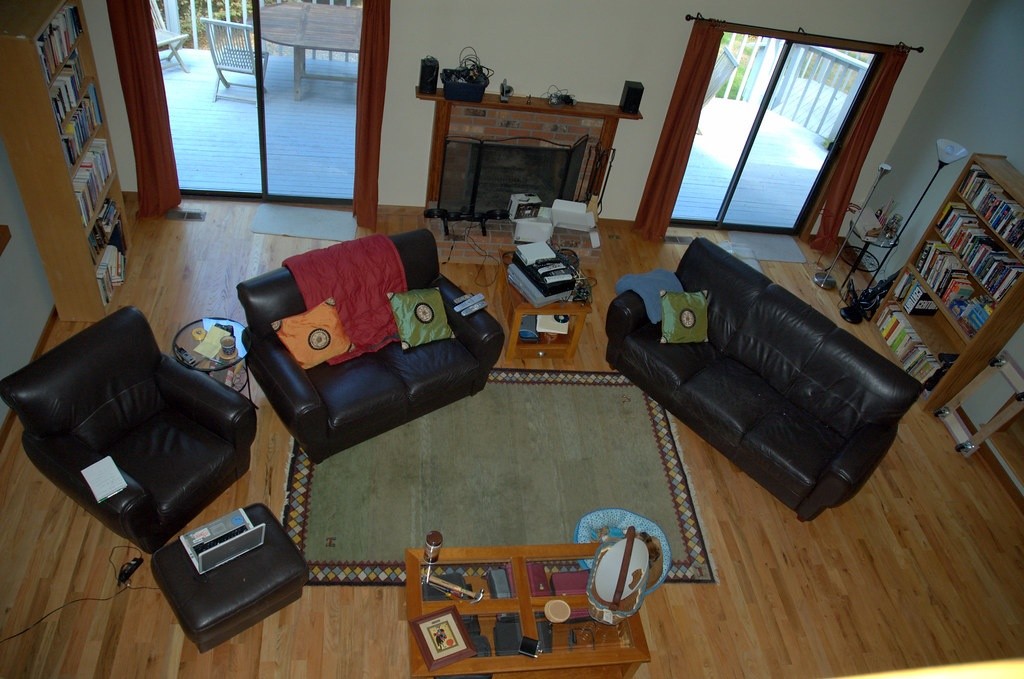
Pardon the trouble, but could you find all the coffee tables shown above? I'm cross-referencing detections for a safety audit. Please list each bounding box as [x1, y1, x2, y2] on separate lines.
[172, 317, 259, 409]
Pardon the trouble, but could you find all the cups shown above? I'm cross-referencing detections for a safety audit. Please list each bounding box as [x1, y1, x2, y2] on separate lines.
[220, 336, 236, 355]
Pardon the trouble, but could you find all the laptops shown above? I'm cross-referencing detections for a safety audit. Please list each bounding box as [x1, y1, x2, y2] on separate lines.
[179, 508, 266, 574]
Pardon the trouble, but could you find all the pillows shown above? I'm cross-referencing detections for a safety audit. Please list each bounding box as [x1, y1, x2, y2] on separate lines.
[387, 287, 457, 352]
[271, 296, 356, 372]
[657, 288, 712, 344]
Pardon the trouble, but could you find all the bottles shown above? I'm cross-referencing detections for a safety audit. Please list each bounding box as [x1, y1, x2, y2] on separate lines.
[423, 531, 443, 564]
[883, 213, 903, 239]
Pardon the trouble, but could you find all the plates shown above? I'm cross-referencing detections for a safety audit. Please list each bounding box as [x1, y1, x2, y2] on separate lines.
[595, 538, 649, 603]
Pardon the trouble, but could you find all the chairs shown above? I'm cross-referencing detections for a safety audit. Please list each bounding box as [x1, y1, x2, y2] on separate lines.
[149, 0, 192, 73]
[199, 17, 269, 107]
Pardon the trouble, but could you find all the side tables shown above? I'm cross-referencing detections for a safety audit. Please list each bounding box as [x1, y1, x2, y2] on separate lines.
[494, 247, 592, 362]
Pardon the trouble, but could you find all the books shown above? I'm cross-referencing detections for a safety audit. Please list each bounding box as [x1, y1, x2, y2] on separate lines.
[38, 5, 128, 307]
[876, 306, 940, 385]
[896, 164, 1024, 332]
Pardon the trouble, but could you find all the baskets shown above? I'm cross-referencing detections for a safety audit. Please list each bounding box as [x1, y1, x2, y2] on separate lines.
[585, 526, 651, 625]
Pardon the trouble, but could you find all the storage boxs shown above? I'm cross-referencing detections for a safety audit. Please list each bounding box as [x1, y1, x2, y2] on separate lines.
[505, 563, 551, 598]
[441, 68, 489, 104]
[536, 315, 569, 335]
[550, 569, 596, 624]
[903, 286, 939, 317]
[506, 193, 543, 220]
[419, 57, 440, 95]
[519, 315, 541, 344]
[537, 206, 552, 219]
[495, 621, 552, 654]
[619, 80, 645, 115]
[433, 614, 492, 679]
[421, 573, 466, 601]
[513, 216, 553, 244]
[552, 199, 596, 232]
[487, 568, 511, 598]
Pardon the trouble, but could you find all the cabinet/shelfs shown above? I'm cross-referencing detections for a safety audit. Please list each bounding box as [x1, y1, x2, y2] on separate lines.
[0, 0, 131, 324]
[405, 541, 652, 679]
[870, 153, 1024, 415]
[823, 218, 900, 306]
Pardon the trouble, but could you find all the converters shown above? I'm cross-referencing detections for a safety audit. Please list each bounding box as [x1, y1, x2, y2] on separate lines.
[118, 558, 144, 583]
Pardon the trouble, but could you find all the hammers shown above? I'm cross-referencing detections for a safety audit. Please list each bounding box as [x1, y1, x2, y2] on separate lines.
[428, 574, 484, 604]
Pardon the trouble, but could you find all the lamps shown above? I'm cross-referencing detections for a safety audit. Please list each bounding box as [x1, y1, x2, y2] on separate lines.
[839, 139, 971, 325]
[811, 162, 893, 291]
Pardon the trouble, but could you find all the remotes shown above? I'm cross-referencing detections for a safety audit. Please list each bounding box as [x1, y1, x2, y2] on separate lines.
[461, 300, 488, 316]
[454, 293, 485, 312]
[453, 292, 475, 304]
[535, 257, 573, 284]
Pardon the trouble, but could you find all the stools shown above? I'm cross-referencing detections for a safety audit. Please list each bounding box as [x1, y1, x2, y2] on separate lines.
[151, 503, 310, 655]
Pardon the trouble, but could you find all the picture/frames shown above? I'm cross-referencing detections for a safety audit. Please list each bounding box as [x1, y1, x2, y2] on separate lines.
[408, 604, 477, 674]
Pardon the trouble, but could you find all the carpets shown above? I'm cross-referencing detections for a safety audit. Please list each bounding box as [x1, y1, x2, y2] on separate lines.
[279, 368, 724, 588]
[718, 242, 764, 274]
[728, 230, 807, 264]
[251, 202, 358, 243]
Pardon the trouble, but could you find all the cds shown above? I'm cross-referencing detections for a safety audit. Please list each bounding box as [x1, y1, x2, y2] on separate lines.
[554, 315, 569, 323]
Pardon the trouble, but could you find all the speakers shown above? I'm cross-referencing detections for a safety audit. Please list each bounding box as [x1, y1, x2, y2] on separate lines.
[419, 59, 439, 94]
[619, 80, 645, 114]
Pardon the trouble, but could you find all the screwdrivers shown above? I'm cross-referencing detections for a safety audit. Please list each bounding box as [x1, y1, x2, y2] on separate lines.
[424, 582, 463, 603]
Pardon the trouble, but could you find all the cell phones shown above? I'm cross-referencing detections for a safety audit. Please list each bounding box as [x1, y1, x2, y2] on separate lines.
[174, 343, 196, 364]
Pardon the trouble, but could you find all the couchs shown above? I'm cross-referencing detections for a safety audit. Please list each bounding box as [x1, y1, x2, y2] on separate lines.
[0, 306, 258, 557]
[233, 227, 506, 467]
[602, 237, 925, 524]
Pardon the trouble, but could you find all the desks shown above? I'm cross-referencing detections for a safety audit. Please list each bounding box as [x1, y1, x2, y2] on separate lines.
[245, 2, 363, 102]
